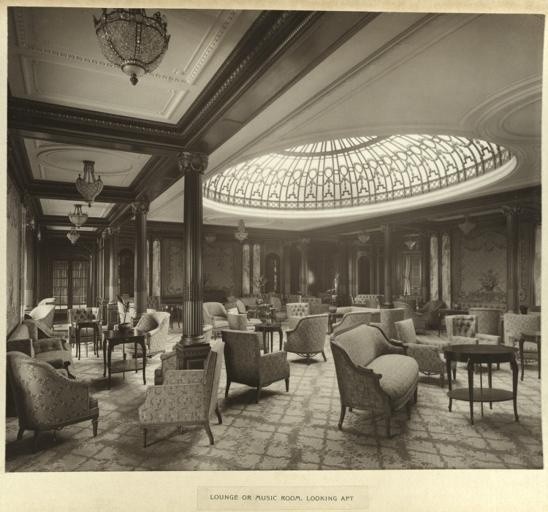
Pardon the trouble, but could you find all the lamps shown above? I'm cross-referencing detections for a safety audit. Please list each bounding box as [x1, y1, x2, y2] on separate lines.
[92, 8, 170, 87]
[361, 230, 373, 245]
[202, 233, 216, 239]
[66, 227, 80, 244]
[456, 210, 476, 234]
[234, 219, 249, 243]
[404, 229, 414, 249]
[67, 205, 88, 229]
[75, 160, 104, 208]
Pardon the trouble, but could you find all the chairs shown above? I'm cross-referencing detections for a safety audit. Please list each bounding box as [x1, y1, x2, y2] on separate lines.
[6, 292, 540, 450]
